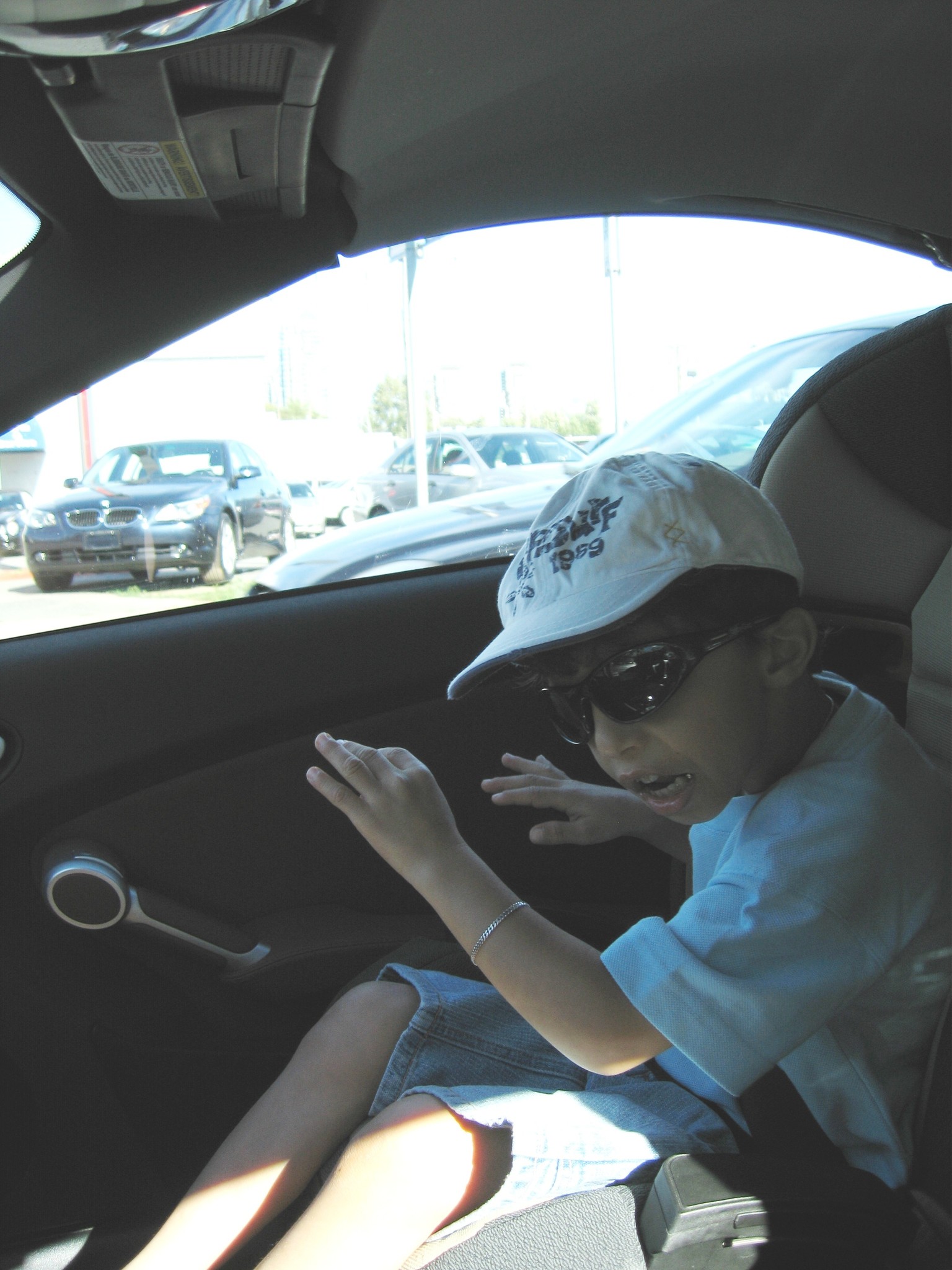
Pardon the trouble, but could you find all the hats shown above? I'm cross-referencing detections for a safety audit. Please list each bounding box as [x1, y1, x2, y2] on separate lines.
[447, 451, 803, 699]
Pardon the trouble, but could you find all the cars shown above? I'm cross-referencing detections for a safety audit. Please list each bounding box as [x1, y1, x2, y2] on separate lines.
[313, 425, 767, 527]
[284, 479, 328, 538]
[0, 489, 39, 558]
[22, 438, 297, 593]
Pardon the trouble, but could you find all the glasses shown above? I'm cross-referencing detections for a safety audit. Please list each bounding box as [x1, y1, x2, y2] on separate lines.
[537, 611, 792, 745]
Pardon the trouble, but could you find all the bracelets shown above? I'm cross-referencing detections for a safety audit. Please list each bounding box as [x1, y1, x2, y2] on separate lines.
[469, 896, 533, 967]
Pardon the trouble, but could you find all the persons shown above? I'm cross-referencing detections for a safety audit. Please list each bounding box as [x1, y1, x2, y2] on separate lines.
[109, 451, 952, 1270]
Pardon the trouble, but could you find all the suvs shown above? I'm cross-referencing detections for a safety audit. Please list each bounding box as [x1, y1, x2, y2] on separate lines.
[248, 298, 948, 602]
